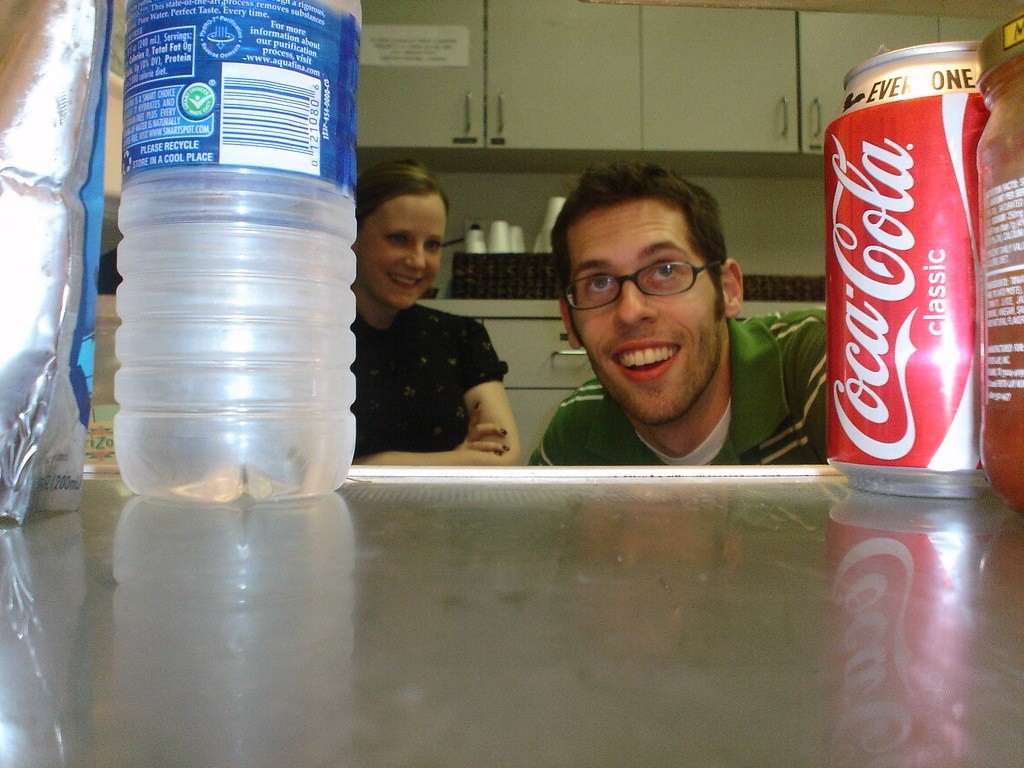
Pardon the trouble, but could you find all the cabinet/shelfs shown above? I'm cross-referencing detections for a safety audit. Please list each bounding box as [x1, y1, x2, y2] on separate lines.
[640, 5, 938, 177]
[356, 0, 638, 175]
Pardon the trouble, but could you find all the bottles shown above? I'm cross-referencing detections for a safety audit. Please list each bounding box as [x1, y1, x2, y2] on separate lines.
[114, 0, 360, 499]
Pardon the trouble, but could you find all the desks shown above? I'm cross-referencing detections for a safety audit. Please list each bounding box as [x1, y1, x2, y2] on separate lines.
[0, 464, 1024, 768]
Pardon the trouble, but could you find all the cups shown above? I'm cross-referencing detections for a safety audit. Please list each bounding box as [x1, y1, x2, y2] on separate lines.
[464, 195, 569, 254]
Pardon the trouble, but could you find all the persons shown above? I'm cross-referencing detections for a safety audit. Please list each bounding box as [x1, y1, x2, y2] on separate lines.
[353, 158, 518, 466]
[529, 162, 827, 465]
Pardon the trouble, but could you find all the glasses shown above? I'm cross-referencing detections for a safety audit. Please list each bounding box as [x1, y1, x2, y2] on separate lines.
[564, 259, 717, 309]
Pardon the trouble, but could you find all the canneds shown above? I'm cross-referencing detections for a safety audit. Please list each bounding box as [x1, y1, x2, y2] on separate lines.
[822, 11, 1024, 518]
